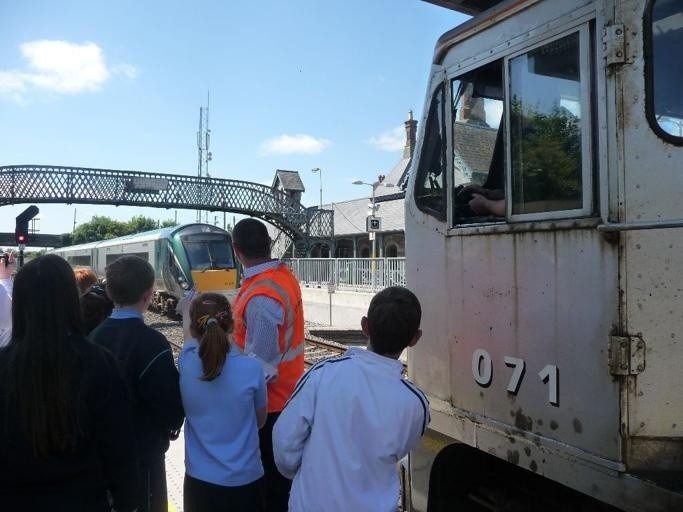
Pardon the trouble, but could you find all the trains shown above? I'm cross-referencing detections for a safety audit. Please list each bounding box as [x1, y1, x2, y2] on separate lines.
[46, 222, 239, 321]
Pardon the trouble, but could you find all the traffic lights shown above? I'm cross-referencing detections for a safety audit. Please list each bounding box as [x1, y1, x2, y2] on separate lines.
[16, 221, 28, 244]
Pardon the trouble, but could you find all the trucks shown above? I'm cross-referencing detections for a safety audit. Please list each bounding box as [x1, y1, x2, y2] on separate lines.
[404, 1, 681, 510]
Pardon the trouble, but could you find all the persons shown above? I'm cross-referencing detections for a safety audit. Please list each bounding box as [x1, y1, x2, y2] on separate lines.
[468, 81, 585, 215]
[87, 255, 183, 512]
[176, 289, 268, 512]
[271, 287, 431, 512]
[1, 252, 114, 347]
[1, 254, 133, 512]
[231, 218, 305, 511]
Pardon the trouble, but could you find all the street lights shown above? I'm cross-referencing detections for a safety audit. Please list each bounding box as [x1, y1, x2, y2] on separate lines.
[350, 175, 395, 292]
[310, 168, 323, 210]
